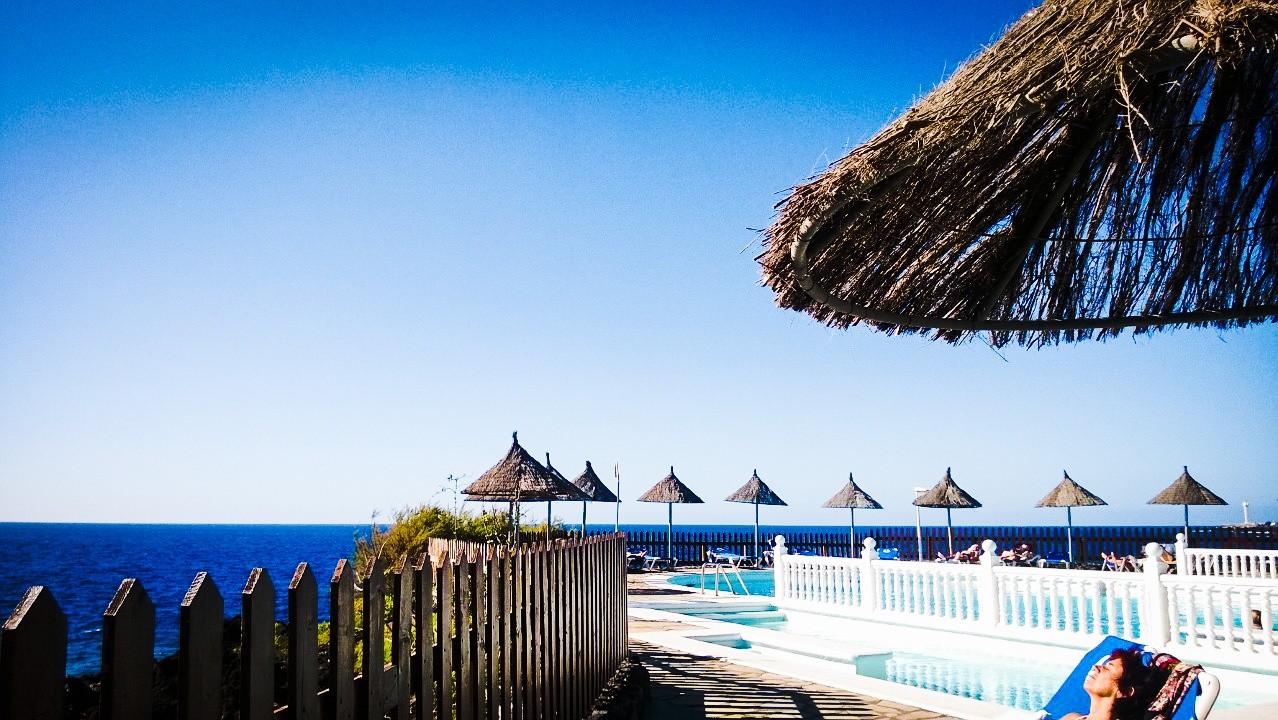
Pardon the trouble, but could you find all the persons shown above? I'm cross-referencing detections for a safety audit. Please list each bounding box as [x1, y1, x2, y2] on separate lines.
[944, 542, 1138, 572]
[626, 549, 648, 558]
[1058, 647, 1158, 720]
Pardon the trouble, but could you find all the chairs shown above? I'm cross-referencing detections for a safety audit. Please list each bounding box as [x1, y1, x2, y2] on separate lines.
[794, 548, 820, 556]
[877, 548, 900, 560]
[626, 548, 673, 570]
[706, 546, 757, 569]
[935, 552, 1145, 573]
[1017, 636, 1221, 720]
